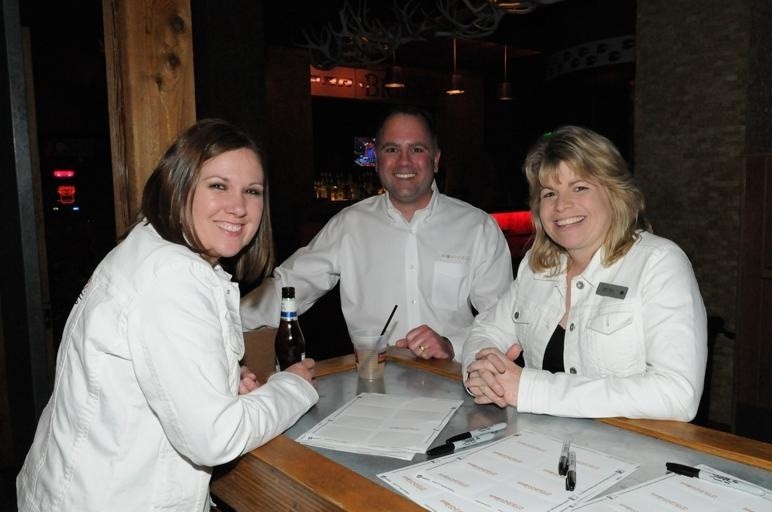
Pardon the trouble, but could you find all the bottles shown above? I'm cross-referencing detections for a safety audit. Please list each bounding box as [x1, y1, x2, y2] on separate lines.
[274, 286, 307, 373]
[316, 169, 379, 202]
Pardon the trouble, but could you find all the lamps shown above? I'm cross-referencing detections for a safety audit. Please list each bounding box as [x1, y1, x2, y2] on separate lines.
[497, 45, 513, 100]
[444, 37, 466, 94]
[385, 40, 406, 88]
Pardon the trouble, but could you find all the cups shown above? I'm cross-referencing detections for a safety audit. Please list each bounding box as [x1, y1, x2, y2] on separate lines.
[349, 327, 389, 380]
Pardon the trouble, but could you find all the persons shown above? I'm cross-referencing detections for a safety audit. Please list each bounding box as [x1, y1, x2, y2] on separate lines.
[14, 118, 319, 510]
[239, 104, 514, 362]
[461, 125, 708, 423]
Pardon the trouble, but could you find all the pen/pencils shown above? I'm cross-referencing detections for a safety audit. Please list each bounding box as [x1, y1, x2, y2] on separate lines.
[565, 450, 577, 490]
[666, 462, 765, 496]
[427, 432, 495, 456]
[558, 442, 570, 474]
[446, 422, 508, 445]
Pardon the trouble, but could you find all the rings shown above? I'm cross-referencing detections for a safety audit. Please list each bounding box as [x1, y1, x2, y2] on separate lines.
[419, 345, 425, 351]
[308, 366, 317, 376]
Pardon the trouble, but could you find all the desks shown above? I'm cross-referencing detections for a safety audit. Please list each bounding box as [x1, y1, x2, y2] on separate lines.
[210, 344, 772, 512]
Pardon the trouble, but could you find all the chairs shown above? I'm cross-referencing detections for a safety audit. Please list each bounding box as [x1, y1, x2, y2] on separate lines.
[688, 313, 725, 427]
